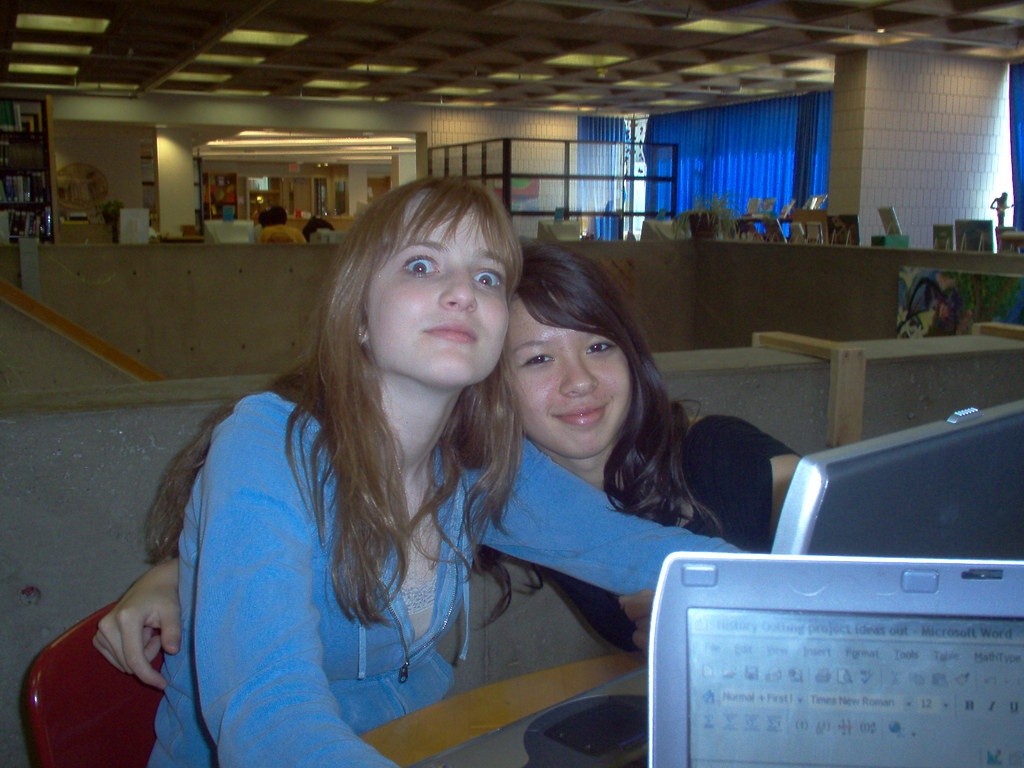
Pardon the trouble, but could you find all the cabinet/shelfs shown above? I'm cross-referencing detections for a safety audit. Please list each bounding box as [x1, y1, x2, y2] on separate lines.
[0, 92, 59, 247]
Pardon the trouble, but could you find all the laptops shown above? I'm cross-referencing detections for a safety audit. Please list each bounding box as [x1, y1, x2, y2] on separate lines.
[524, 398, 1024, 768]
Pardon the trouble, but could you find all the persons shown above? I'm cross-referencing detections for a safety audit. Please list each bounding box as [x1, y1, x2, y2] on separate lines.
[93, 236, 804, 692]
[147, 173, 743, 768]
[254, 206, 307, 245]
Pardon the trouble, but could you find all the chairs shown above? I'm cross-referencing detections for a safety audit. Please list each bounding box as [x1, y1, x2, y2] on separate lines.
[28, 602, 165, 768]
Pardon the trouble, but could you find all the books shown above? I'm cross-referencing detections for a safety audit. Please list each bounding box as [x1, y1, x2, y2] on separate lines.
[0, 102, 53, 243]
